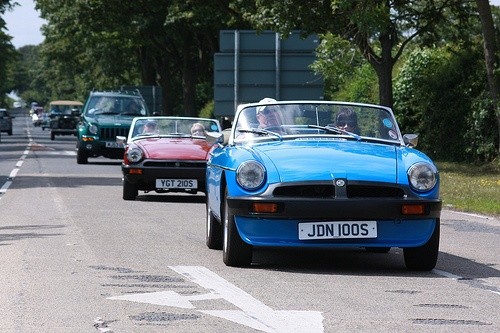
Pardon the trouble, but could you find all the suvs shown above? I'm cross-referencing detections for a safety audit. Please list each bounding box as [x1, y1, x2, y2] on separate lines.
[71, 88, 159, 164]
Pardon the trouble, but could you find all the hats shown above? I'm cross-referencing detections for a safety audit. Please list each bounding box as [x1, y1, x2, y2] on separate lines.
[256, 98, 280, 114]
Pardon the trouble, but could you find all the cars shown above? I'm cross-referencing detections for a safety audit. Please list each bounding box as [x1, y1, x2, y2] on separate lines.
[204, 98, 444, 271]
[28, 98, 84, 141]
[115, 115, 224, 201]
[0, 108, 16, 136]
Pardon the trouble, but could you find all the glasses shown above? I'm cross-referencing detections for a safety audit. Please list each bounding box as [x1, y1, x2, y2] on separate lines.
[337, 120, 355, 126]
[146, 123, 156, 127]
[261, 108, 279, 115]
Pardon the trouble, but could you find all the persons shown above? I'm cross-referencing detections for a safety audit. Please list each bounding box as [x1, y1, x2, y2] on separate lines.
[191, 123, 206, 136]
[254, 98, 296, 137]
[144, 120, 156, 134]
[336, 108, 357, 136]
